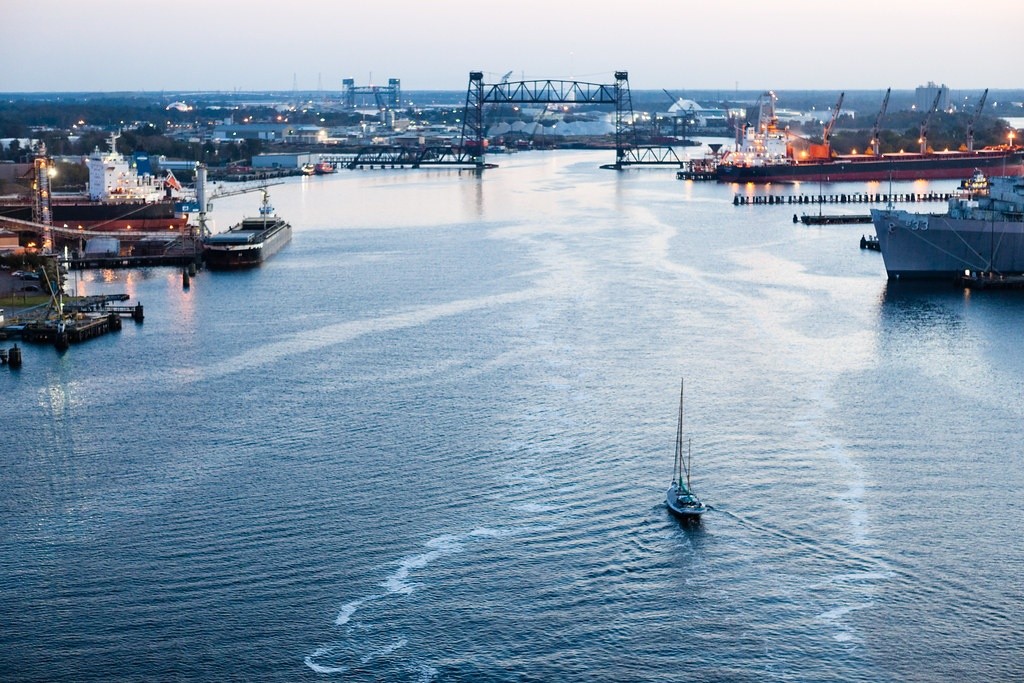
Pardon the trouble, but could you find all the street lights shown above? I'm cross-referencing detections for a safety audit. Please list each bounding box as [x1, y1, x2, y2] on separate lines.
[1006, 131, 1016, 146]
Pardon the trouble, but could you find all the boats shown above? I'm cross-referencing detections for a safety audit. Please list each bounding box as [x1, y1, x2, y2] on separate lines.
[868, 153, 1024, 288]
[718, 85, 1024, 184]
[201, 189, 294, 267]
[665, 377, 707, 515]
